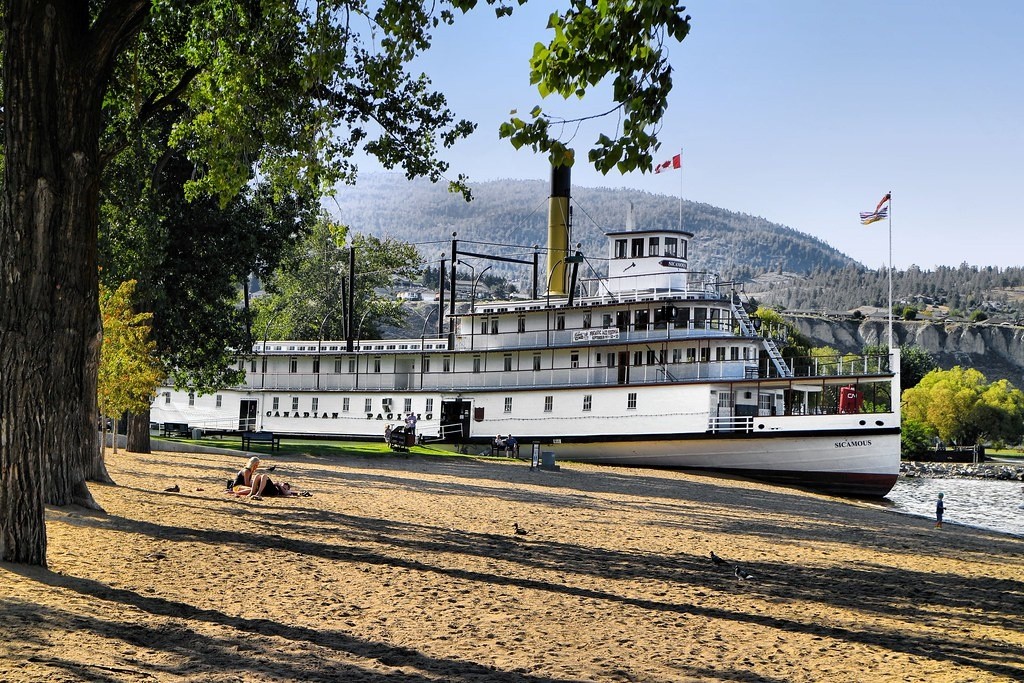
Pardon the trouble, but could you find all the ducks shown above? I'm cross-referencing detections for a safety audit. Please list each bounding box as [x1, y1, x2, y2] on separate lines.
[511, 522, 529, 536]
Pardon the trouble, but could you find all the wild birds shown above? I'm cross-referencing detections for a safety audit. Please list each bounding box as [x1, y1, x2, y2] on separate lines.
[163, 483, 182, 493]
[707, 549, 733, 568]
[733, 564, 754, 583]
[265, 464, 278, 472]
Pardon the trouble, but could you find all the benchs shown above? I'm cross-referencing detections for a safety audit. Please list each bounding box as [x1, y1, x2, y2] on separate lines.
[164, 421, 193, 439]
[491, 437, 519, 458]
[241, 431, 280, 452]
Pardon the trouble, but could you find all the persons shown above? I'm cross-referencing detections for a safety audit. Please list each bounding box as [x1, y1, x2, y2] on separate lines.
[934, 493, 946, 529]
[502, 433, 517, 459]
[495, 434, 504, 446]
[247, 474, 290, 499]
[385, 425, 391, 444]
[405, 412, 417, 435]
[973, 443, 985, 463]
[234, 457, 260, 487]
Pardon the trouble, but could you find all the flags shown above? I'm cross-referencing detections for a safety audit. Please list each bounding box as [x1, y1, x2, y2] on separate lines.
[860, 195, 890, 224]
[654, 153, 680, 174]
